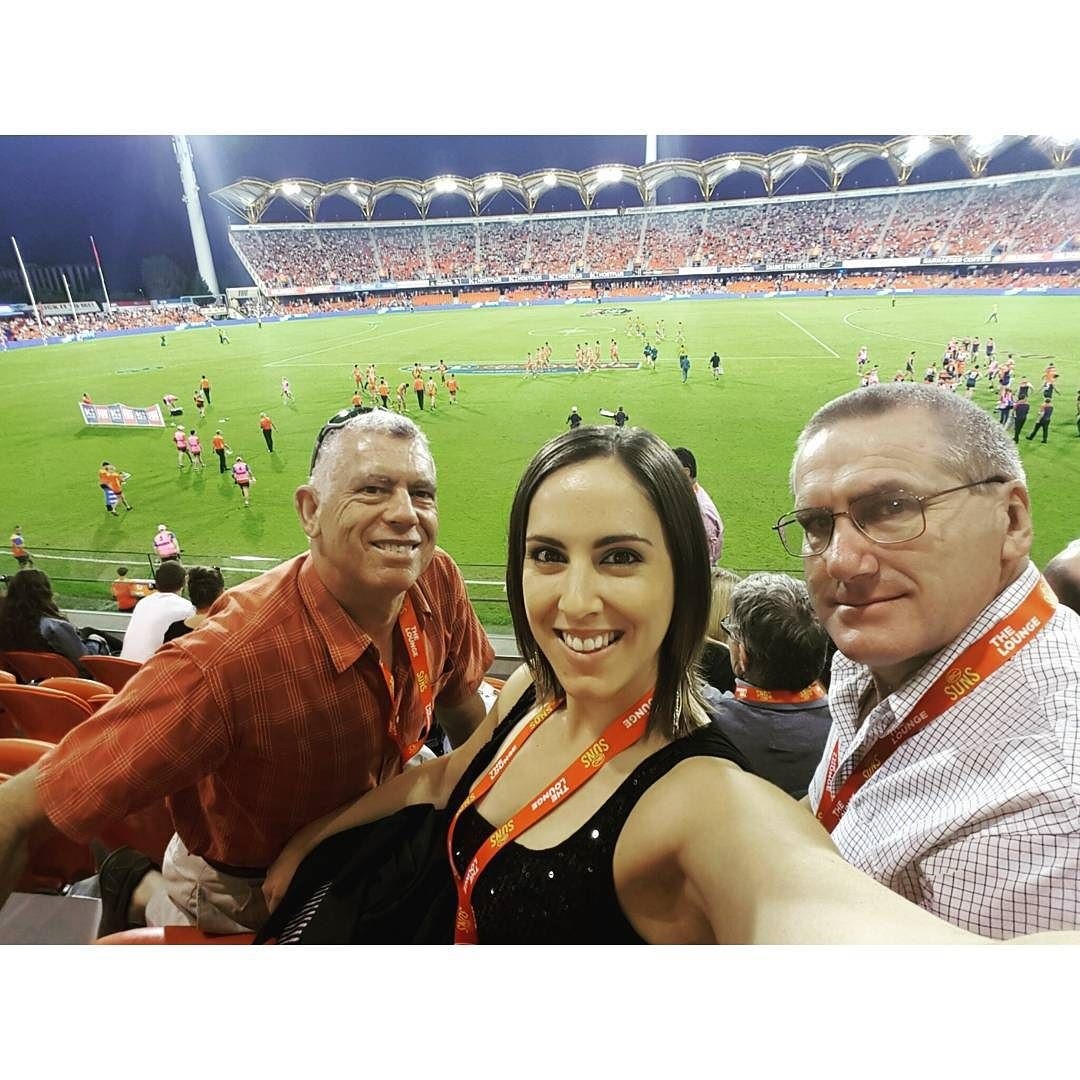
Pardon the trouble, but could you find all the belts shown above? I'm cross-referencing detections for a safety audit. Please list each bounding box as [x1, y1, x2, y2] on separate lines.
[201, 857, 269, 879]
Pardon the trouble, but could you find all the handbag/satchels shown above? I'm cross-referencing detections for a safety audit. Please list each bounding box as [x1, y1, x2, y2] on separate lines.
[275, 803, 457, 945]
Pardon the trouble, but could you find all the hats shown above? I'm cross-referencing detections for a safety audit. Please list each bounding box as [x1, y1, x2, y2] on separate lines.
[177, 424, 185, 429]
[236, 457, 242, 460]
[157, 524, 168, 532]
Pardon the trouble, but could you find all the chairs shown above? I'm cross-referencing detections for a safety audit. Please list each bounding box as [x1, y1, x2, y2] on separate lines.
[78, 654, 143, 694]
[0, 683, 95, 744]
[0, 738, 58, 775]
[0, 651, 82, 682]
[38, 676, 114, 701]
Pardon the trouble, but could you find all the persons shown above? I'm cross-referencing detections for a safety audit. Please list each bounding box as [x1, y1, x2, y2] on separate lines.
[261, 424, 1080, 945]
[0, 171, 1080, 945]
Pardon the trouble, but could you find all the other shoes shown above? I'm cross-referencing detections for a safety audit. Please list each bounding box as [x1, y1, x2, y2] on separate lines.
[99, 845, 165, 935]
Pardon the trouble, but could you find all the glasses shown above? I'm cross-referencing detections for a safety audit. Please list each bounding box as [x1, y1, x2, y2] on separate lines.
[765, 473, 1009, 560]
[308, 407, 375, 472]
[720, 614, 738, 638]
